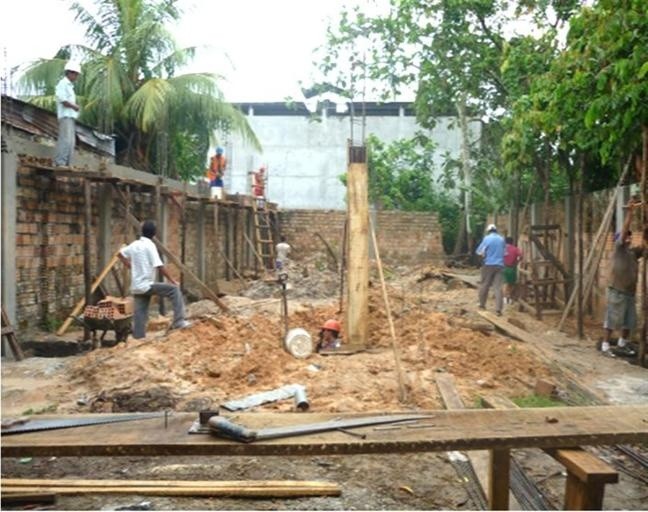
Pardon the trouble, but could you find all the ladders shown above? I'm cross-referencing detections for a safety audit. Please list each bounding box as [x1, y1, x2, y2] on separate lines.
[250, 171, 276, 271]
[517, 224, 568, 321]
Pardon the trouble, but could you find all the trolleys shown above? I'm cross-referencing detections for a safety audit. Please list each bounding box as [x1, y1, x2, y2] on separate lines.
[67, 310, 135, 350]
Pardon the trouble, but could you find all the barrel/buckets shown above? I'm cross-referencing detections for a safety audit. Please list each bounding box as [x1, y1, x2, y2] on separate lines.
[284, 327, 313, 359]
[256, 196, 264, 208]
[276, 259, 282, 268]
[210, 186, 222, 199]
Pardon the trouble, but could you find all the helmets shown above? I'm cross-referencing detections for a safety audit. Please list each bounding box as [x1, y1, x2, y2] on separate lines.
[215, 145, 225, 154]
[64, 61, 84, 76]
[319, 318, 342, 335]
[486, 223, 496, 232]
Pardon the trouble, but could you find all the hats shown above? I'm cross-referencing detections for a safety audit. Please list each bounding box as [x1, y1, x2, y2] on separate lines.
[614, 228, 633, 242]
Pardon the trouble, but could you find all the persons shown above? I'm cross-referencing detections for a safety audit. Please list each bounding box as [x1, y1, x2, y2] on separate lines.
[501, 237, 525, 305]
[248, 167, 268, 196]
[117, 221, 193, 340]
[315, 318, 344, 353]
[50, 58, 86, 168]
[601, 196, 644, 357]
[275, 236, 293, 271]
[474, 224, 508, 316]
[205, 146, 227, 187]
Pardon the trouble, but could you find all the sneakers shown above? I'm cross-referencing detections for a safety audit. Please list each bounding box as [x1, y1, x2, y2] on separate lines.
[172, 319, 192, 329]
[475, 296, 514, 317]
[614, 343, 637, 358]
[601, 347, 616, 359]
